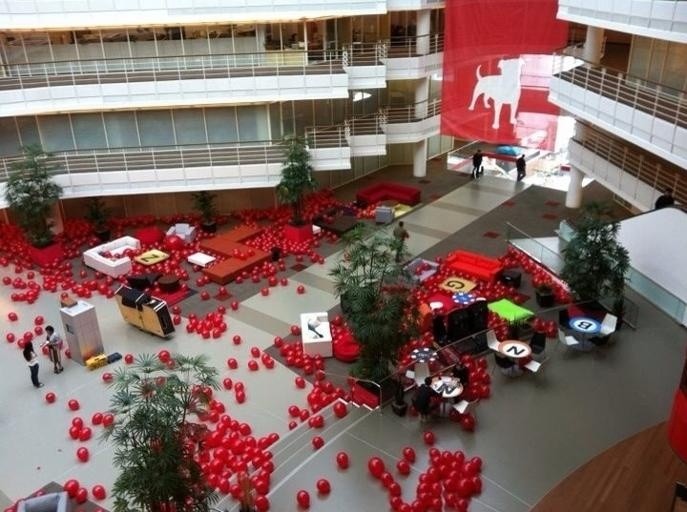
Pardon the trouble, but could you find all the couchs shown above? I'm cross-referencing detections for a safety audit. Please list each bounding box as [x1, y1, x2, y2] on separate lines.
[167, 226, 196, 244]
[377, 257, 440, 303]
[300, 312, 333, 359]
[83, 235, 142, 279]
[444, 250, 505, 286]
[356, 181, 422, 211]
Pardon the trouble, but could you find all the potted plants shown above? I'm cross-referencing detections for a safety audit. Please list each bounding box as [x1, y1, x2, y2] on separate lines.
[1, 143, 63, 247]
[273, 133, 321, 227]
[561, 197, 630, 322]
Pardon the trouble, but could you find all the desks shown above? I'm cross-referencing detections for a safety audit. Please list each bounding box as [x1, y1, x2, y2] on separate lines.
[536, 289, 555, 308]
[502, 270, 522, 289]
[157, 275, 179, 292]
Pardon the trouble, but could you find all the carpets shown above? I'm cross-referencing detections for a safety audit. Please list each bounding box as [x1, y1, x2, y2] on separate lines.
[263, 183, 633, 399]
[0, 137, 497, 322]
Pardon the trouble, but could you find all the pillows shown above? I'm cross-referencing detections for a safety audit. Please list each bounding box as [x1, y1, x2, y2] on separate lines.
[309, 316, 321, 328]
[175, 223, 190, 234]
[308, 330, 318, 339]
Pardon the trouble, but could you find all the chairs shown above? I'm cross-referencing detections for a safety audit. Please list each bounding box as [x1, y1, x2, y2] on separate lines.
[412, 362, 479, 419]
[126, 272, 163, 291]
[557, 312, 618, 357]
[486, 329, 550, 387]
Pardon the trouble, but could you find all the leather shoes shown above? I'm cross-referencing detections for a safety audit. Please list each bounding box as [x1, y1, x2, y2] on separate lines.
[34, 367, 63, 388]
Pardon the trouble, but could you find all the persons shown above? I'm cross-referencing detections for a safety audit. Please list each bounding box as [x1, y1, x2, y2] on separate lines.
[472, 149, 482, 176]
[515, 154, 526, 183]
[656, 187, 673, 210]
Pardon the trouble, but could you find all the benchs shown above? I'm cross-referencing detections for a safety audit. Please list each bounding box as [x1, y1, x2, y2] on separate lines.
[312, 207, 359, 238]
